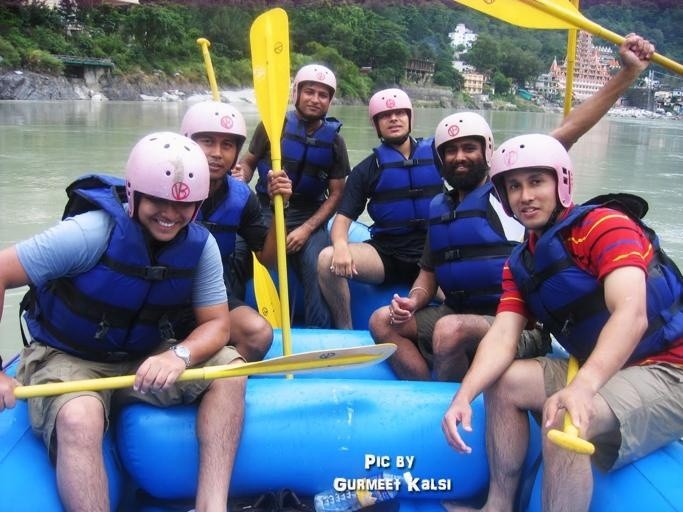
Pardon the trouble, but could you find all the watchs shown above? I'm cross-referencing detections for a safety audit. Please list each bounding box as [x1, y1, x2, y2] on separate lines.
[169, 344, 191, 367]
[269, 201, 289, 218]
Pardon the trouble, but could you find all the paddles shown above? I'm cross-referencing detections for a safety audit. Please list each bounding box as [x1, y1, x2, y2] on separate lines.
[454, 0, 683, 75]
[9, 341, 400, 401]
[196, 37, 284, 331]
[249, 7, 292, 384]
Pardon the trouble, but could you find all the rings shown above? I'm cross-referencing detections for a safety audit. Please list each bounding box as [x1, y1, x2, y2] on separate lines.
[330, 266, 332, 269]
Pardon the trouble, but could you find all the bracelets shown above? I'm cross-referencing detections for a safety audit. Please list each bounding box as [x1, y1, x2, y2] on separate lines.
[408, 286, 430, 299]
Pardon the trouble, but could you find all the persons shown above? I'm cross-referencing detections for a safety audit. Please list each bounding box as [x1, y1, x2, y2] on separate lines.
[442, 132, 683, 512]
[0, 132, 248, 512]
[317, 86, 447, 329]
[230, 64, 353, 329]
[179, 100, 293, 378]
[368, 32, 654, 383]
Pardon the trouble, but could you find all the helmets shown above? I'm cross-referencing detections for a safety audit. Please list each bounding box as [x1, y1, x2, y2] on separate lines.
[489, 133, 573, 215]
[125, 130, 210, 224]
[368, 87, 413, 133]
[181, 102, 247, 170]
[433, 106, 496, 167]
[293, 65, 336, 103]
